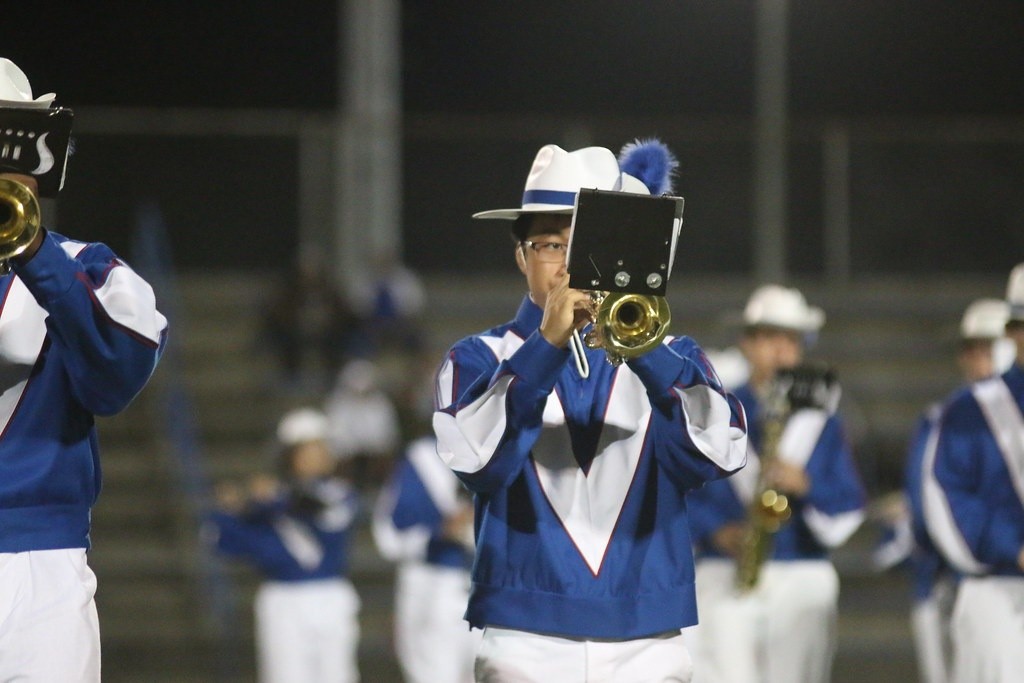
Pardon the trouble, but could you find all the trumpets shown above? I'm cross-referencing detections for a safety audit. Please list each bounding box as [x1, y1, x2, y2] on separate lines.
[0, 175, 42, 262]
[583, 288, 671, 368]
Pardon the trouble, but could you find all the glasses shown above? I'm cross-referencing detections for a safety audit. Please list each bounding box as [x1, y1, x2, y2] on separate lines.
[524, 241, 567, 263]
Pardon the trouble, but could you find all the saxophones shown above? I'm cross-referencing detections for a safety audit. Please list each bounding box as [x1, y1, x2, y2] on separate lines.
[733, 372, 794, 591]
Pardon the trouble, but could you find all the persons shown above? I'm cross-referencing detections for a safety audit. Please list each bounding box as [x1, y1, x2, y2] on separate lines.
[682, 284, 867, 683]
[872, 264, 1024, 683]
[328, 229, 427, 480]
[433, 143, 749, 683]
[371, 436, 481, 683]
[208, 409, 362, 683]
[0, 57, 168, 683]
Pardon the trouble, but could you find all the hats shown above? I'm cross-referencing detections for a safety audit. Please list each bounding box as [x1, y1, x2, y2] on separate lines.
[278, 409, 333, 446]
[743, 285, 827, 331]
[1006, 263, 1024, 322]
[959, 298, 1012, 338]
[472, 144, 651, 221]
[0, 58, 56, 138]
